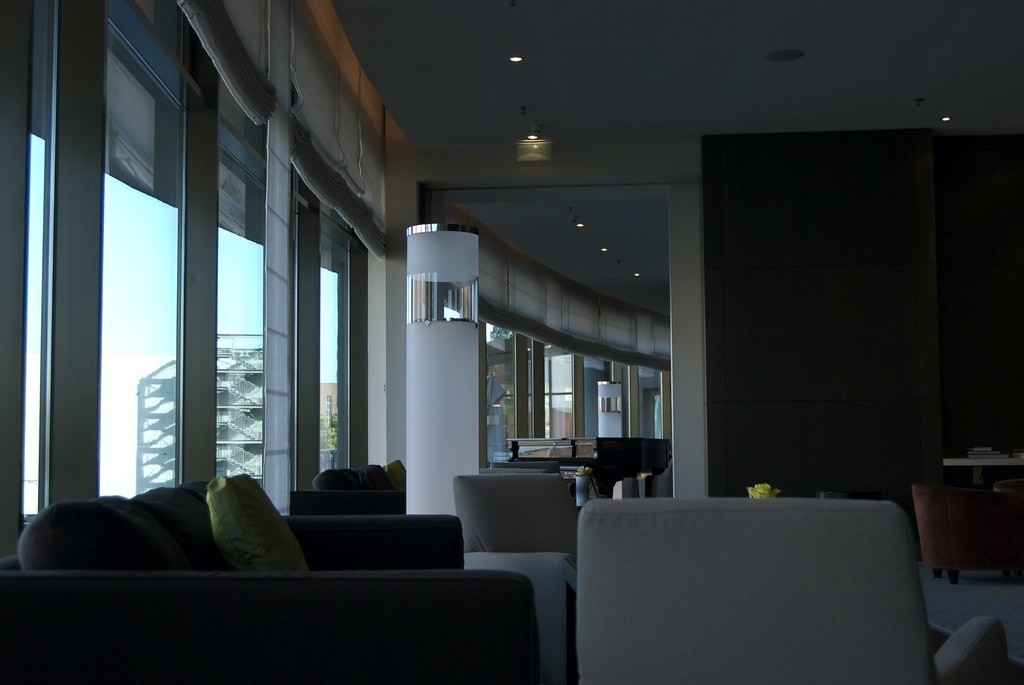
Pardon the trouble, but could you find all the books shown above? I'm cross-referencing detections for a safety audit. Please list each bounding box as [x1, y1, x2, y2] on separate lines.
[967, 445, 1024, 458]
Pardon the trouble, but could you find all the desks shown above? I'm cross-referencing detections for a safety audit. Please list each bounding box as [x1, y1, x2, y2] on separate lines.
[939, 456, 1024, 483]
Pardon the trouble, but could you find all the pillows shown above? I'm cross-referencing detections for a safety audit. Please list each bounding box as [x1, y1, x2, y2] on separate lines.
[206, 474, 308, 574]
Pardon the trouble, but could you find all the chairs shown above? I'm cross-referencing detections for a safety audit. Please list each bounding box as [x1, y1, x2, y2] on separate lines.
[453, 472, 578, 553]
[910, 484, 1024, 585]
[577, 497, 1007, 685]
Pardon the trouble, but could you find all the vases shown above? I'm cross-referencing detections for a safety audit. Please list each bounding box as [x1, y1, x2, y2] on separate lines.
[575, 477, 589, 508]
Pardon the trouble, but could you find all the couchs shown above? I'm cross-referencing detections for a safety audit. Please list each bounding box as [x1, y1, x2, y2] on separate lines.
[289, 464, 406, 518]
[0, 480, 538, 685]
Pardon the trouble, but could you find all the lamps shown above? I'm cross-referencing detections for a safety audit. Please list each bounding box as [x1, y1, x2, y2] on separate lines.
[527, 124, 544, 141]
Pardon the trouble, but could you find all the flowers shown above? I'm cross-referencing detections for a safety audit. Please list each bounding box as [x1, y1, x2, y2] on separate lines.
[749, 483, 780, 501]
[572, 467, 592, 476]
